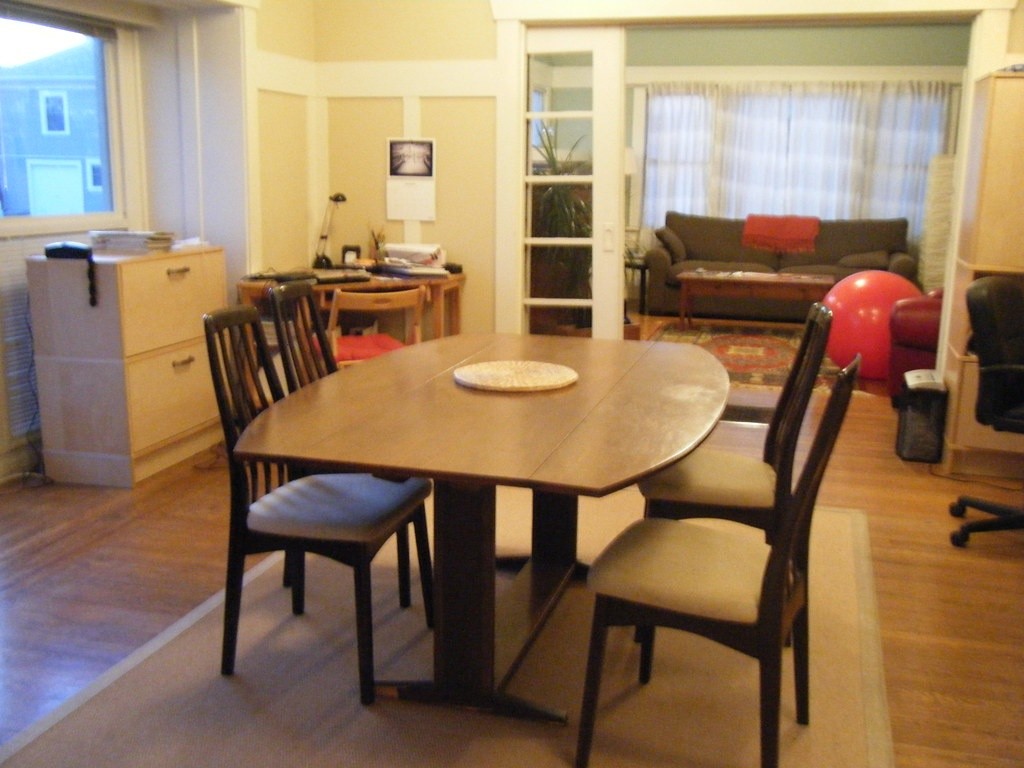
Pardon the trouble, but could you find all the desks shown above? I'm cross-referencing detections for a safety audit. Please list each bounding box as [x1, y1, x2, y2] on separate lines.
[237, 272, 466, 407]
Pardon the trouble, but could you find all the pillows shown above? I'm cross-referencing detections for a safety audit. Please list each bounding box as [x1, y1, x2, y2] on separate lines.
[840, 251, 888, 269]
[655, 227, 687, 263]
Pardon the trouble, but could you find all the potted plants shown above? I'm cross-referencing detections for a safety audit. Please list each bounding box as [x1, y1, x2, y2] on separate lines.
[533, 121, 592, 329]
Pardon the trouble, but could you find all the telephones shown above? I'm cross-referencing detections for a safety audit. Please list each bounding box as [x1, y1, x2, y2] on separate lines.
[45, 240, 93, 259]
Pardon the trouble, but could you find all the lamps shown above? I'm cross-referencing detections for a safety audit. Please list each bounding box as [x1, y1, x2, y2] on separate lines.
[312, 193, 346, 269]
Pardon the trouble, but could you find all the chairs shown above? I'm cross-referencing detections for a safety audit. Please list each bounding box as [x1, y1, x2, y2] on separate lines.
[203, 305, 433, 706]
[577, 353, 861, 768]
[268, 281, 411, 616]
[633, 302, 835, 688]
[949, 275, 1024, 549]
[303, 285, 426, 371]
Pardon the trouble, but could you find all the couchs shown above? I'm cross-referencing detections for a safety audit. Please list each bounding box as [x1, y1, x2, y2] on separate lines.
[644, 210, 916, 324]
[887, 287, 943, 406]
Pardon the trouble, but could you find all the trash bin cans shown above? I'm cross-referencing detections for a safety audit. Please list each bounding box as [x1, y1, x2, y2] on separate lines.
[894, 369, 947, 464]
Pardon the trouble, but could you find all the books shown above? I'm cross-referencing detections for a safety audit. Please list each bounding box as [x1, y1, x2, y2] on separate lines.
[90, 229, 175, 254]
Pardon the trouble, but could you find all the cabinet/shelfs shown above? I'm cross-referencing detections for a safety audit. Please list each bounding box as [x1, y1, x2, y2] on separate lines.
[931, 70, 1024, 479]
[25, 243, 228, 486]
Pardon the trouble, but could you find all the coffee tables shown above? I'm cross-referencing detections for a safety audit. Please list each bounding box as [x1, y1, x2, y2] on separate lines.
[676, 270, 836, 331]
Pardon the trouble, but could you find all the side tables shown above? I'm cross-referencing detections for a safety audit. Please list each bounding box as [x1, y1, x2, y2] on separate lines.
[624, 256, 649, 314]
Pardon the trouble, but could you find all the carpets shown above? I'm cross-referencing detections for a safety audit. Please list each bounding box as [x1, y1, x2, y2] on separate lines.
[646, 321, 840, 394]
[0, 508, 901, 768]
